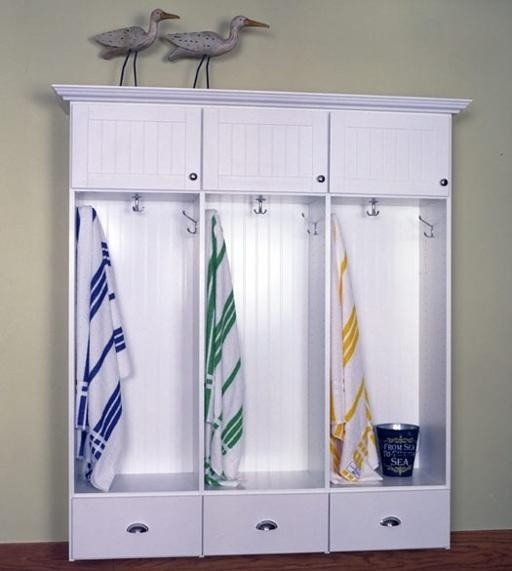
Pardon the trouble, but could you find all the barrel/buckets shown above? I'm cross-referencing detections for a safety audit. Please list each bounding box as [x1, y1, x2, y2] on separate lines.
[376, 422, 421, 477]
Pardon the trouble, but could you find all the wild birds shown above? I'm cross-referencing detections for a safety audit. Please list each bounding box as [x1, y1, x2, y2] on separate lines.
[157, 16, 270, 89]
[87, 9, 180, 87]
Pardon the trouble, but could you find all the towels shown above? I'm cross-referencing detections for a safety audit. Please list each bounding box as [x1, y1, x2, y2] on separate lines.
[77, 202, 135, 492]
[206, 209, 246, 488]
[327, 216, 379, 486]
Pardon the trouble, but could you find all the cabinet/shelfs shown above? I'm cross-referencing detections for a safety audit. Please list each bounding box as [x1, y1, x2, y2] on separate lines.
[44, 82, 473, 561]
